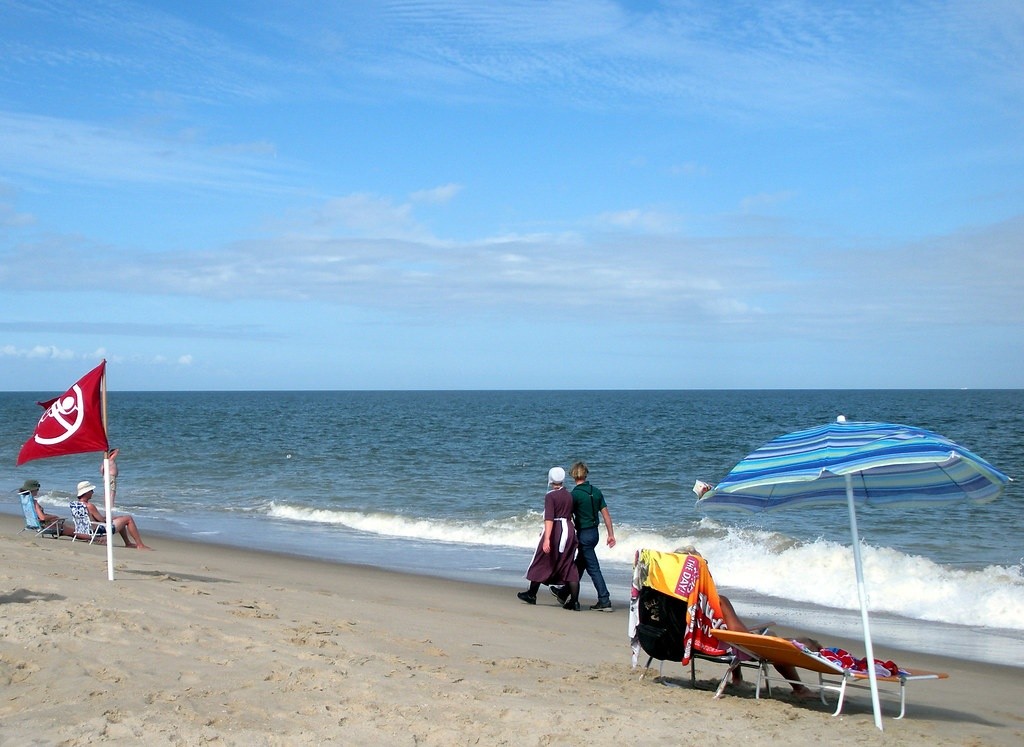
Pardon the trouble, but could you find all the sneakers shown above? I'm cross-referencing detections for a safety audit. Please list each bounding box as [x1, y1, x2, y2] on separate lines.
[590, 600, 613, 611]
[549, 586, 567, 603]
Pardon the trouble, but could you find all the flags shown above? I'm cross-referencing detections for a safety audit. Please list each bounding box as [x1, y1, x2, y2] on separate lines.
[17, 360, 108, 465]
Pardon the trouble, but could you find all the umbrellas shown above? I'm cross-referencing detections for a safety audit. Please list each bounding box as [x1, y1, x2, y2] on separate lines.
[696, 414, 1011, 732]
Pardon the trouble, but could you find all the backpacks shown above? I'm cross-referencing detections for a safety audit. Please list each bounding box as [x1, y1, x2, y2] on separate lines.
[636, 587, 689, 662]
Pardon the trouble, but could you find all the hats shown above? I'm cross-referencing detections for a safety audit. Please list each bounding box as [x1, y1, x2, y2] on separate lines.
[21, 479, 40, 490]
[548, 467, 566, 486]
[77, 481, 96, 496]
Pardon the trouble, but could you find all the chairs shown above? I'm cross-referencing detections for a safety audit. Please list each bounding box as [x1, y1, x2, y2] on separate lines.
[633, 549, 772, 698]
[708, 628, 948, 719]
[16, 490, 70, 539]
[68, 500, 106, 546]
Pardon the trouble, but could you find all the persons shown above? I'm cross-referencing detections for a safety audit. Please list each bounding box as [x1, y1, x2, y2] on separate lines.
[101, 448, 119, 505]
[77, 481, 152, 551]
[549, 462, 615, 612]
[674, 546, 824, 698]
[518, 467, 580, 610]
[20, 480, 106, 543]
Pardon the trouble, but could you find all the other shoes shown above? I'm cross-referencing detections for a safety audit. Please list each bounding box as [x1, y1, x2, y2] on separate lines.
[518, 591, 536, 605]
[563, 600, 581, 611]
